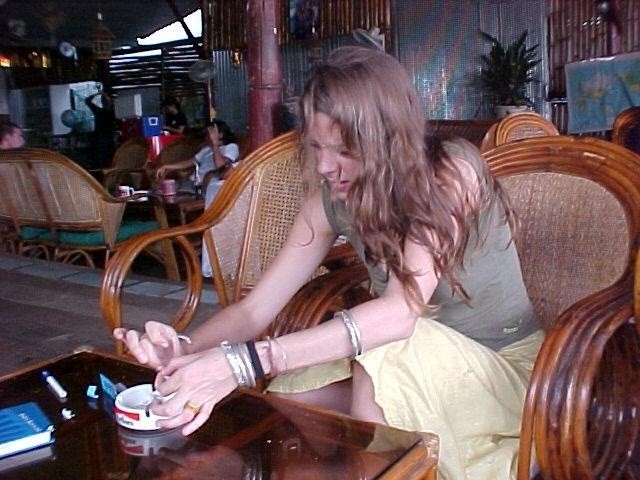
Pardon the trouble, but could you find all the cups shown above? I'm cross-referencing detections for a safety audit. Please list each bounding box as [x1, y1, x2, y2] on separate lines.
[116, 186, 134, 196]
[162, 179, 176, 196]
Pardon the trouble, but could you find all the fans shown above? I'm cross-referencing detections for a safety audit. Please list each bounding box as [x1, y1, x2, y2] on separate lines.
[187, 60, 218, 84]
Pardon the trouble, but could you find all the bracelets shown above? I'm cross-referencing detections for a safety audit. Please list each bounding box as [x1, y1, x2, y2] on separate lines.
[220, 336, 288, 388]
[178, 335, 191, 344]
[333, 309, 363, 360]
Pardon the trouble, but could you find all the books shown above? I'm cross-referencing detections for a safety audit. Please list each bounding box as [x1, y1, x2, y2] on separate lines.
[0, 402, 55, 459]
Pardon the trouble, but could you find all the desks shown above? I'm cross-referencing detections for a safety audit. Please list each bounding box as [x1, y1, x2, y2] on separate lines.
[2, 342, 441, 467]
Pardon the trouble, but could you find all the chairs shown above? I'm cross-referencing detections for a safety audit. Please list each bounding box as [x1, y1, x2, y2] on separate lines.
[2, 112, 254, 280]
[92, 126, 636, 475]
[478, 112, 638, 156]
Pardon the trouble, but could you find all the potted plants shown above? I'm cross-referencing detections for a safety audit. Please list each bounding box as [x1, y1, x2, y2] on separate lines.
[462, 26, 543, 120]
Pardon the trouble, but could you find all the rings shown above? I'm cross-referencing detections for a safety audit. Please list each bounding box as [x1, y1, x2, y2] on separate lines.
[185, 402, 200, 414]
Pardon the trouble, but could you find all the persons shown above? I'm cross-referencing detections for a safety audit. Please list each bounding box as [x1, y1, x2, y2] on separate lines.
[0, 122, 26, 150]
[156, 120, 239, 196]
[162, 96, 188, 133]
[113, 47, 546, 480]
[85, 91, 115, 168]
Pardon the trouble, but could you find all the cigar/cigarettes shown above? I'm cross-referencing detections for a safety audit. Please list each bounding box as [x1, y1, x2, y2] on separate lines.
[40, 370, 68, 398]
